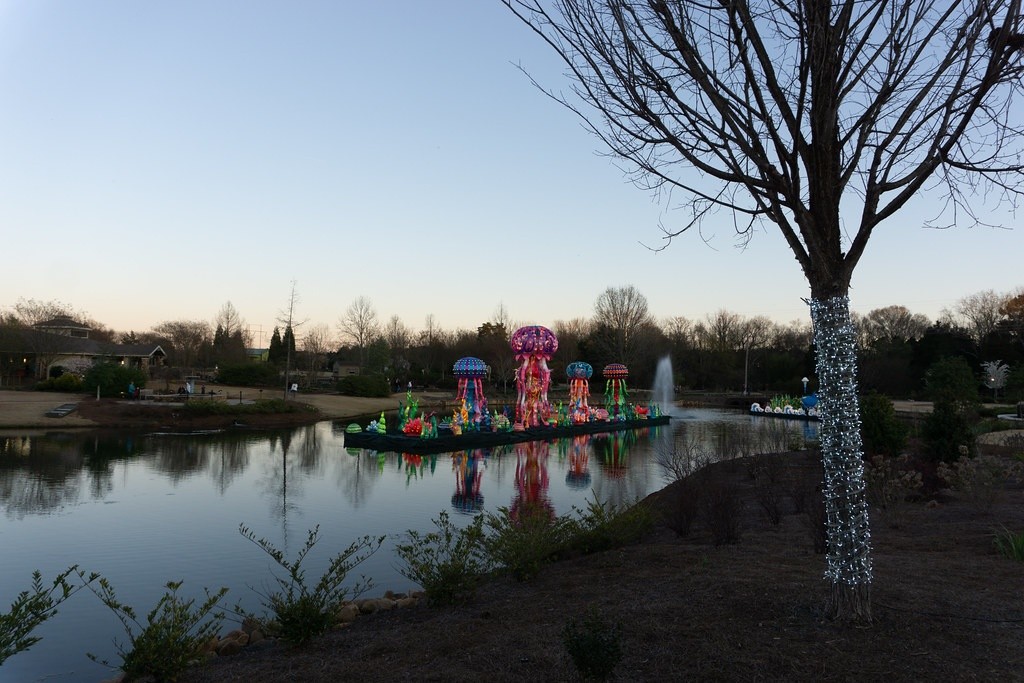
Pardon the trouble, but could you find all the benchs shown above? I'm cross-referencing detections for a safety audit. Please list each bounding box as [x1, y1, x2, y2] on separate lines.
[118, 388, 156, 401]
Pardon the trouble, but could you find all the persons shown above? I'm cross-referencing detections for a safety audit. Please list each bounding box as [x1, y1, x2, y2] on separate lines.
[673, 384, 682, 395]
[742, 383, 747, 396]
[386, 377, 413, 392]
[291, 382, 298, 398]
[179, 385, 217, 394]
[128, 381, 139, 400]
